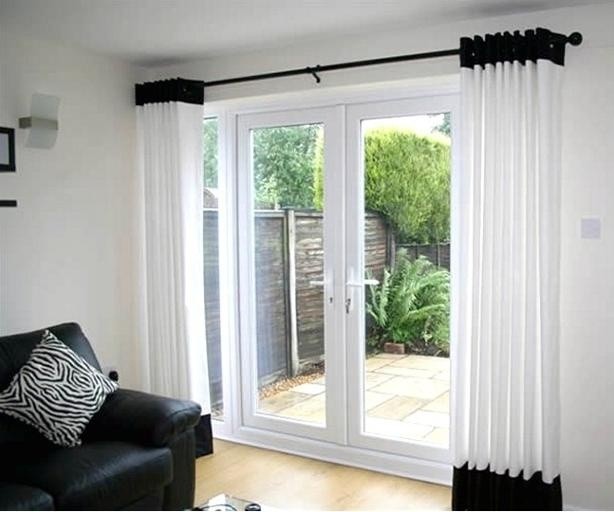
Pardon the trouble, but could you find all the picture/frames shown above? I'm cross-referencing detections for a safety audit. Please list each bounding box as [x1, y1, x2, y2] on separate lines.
[0, 126, 15, 172]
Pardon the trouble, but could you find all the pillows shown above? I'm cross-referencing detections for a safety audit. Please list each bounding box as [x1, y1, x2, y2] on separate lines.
[0, 330, 120, 448]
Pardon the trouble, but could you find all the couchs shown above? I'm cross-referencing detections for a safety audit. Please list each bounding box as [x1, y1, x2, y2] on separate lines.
[0, 322, 202, 511]
